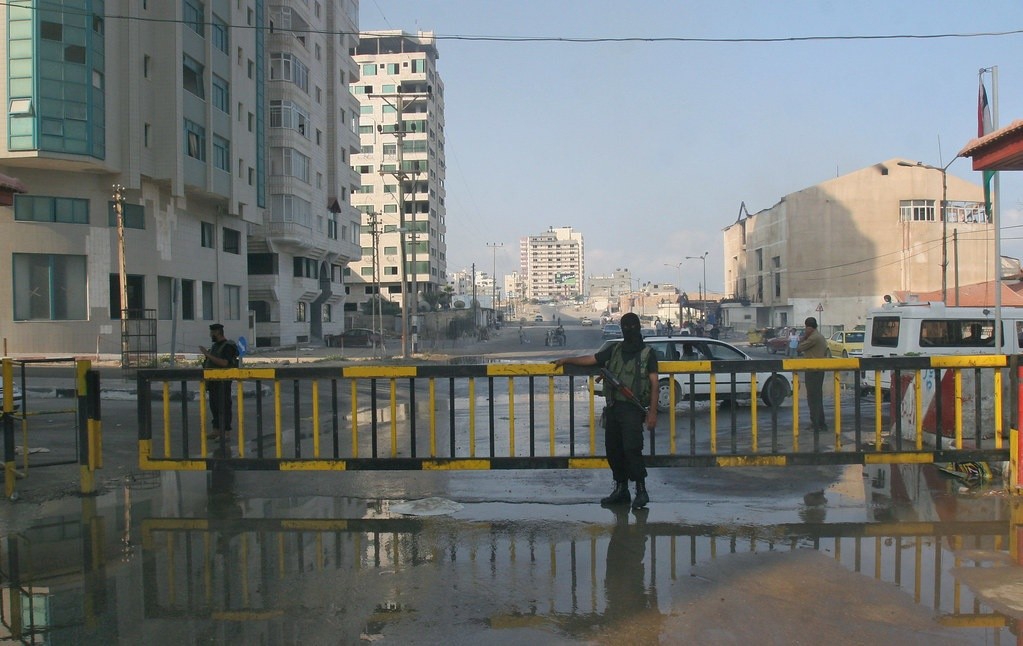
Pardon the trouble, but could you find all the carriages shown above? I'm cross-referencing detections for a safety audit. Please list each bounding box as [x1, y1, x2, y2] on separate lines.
[545, 329, 564, 347]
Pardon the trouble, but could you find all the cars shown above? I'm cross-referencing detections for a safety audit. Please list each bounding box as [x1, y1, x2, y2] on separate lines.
[585, 334, 799, 413]
[641, 328, 657, 337]
[534, 315, 543, 322]
[766, 326, 806, 355]
[825, 330, 864, 359]
[601, 324, 621, 339]
[549, 302, 555, 307]
[582, 318, 592, 327]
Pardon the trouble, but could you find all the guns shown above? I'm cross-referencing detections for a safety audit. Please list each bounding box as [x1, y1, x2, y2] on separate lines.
[595, 366, 650, 415]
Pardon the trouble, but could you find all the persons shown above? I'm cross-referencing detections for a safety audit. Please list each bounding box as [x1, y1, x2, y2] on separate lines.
[563, 503, 661, 646]
[788, 328, 800, 359]
[549, 313, 659, 509]
[680, 343, 694, 361]
[796, 317, 828, 432]
[963, 323, 982, 346]
[556, 324, 567, 346]
[654, 317, 720, 354]
[517, 326, 526, 344]
[198, 324, 240, 442]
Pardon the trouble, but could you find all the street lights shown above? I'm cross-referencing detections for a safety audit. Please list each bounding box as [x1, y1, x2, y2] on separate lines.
[898, 150, 962, 306]
[629, 278, 640, 315]
[685, 252, 709, 323]
[664, 262, 683, 328]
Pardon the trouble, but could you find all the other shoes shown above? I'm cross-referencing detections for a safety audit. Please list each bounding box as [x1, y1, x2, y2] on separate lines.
[633, 490, 650, 508]
[601, 489, 630, 503]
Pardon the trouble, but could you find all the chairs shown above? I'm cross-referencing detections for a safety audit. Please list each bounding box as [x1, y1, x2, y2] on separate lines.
[657, 345, 693, 359]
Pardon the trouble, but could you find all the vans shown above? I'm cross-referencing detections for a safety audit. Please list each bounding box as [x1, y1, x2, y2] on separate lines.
[862, 294, 1023, 400]
